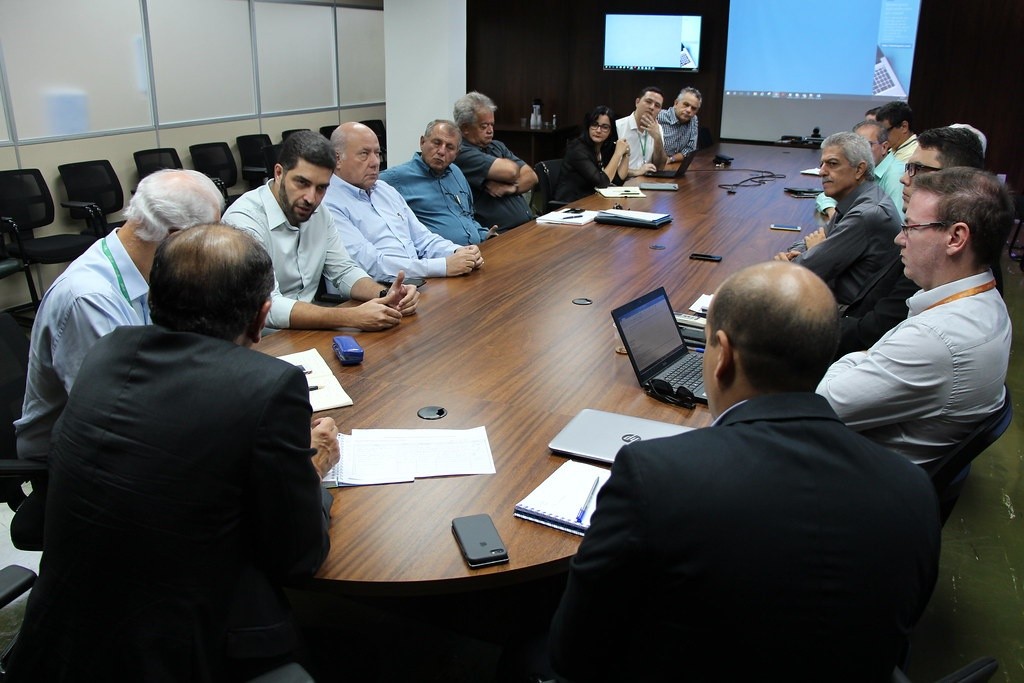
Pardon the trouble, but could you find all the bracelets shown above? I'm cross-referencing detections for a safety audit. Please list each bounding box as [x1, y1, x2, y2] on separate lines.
[513, 182, 518, 193]
[380, 289, 388, 297]
[670, 156, 675, 162]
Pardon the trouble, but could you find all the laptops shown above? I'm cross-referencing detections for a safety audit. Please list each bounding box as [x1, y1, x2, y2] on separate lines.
[680, 43, 695, 68]
[611, 286, 709, 404]
[645, 148, 699, 178]
[548, 409, 697, 465]
[872, 45, 906, 96]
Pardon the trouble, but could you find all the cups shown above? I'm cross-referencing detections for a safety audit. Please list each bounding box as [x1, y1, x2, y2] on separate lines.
[520, 118, 527, 128]
[544, 122, 551, 130]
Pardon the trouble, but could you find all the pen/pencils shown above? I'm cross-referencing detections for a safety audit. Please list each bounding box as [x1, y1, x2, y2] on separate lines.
[621, 193, 640, 195]
[684, 339, 706, 346]
[614, 142, 629, 150]
[563, 215, 583, 219]
[308, 385, 324, 391]
[686, 346, 706, 353]
[577, 477, 600, 523]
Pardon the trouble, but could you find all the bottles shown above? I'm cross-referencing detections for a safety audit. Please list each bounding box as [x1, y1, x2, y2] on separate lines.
[552, 114, 558, 129]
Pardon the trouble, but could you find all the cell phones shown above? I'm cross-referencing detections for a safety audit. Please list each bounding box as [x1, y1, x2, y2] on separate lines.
[689, 253, 722, 262]
[770, 224, 801, 231]
[294, 363, 313, 374]
[452, 514, 509, 567]
[376, 273, 425, 288]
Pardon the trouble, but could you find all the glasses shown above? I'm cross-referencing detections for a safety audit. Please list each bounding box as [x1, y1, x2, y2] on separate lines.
[589, 120, 611, 133]
[445, 191, 476, 217]
[905, 162, 943, 177]
[646, 377, 697, 410]
[901, 222, 957, 239]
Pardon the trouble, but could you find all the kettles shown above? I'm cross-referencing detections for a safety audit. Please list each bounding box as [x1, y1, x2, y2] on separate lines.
[529, 104, 542, 130]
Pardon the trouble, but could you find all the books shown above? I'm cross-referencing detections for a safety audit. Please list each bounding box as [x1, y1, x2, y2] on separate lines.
[536, 208, 598, 225]
[514, 459, 612, 537]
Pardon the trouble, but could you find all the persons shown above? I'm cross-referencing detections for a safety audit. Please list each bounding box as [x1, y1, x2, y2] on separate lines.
[322, 121, 484, 294]
[13, 169, 226, 459]
[378, 120, 498, 245]
[222, 130, 421, 337]
[834, 126, 984, 363]
[547, 260, 942, 683]
[815, 101, 920, 223]
[555, 105, 630, 210]
[615, 86, 667, 178]
[453, 92, 539, 235]
[656, 86, 702, 164]
[774, 130, 905, 318]
[817, 166, 1016, 466]
[5, 221, 341, 683]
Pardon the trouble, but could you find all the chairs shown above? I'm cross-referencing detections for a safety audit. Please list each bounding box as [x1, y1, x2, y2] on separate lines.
[0, 120, 386, 683]
[928, 382, 1013, 530]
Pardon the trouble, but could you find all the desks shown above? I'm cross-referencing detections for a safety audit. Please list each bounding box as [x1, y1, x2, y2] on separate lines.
[249, 143, 831, 683]
[494, 125, 577, 167]
